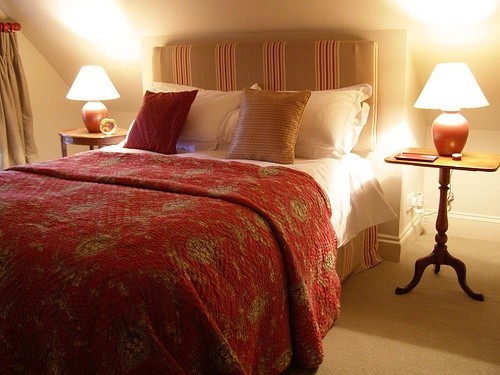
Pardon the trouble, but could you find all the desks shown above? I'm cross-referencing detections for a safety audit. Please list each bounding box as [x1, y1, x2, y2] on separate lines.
[58, 130, 127, 158]
[384, 147, 500, 301]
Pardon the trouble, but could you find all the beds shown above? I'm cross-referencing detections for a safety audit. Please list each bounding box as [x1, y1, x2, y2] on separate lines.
[0, 33, 410, 374]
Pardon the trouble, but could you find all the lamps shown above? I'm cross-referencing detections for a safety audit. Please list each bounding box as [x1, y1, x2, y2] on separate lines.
[413, 62, 495, 156]
[64, 65, 122, 132]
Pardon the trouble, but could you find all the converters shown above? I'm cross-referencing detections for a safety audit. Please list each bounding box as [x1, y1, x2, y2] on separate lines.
[417, 193, 424, 209]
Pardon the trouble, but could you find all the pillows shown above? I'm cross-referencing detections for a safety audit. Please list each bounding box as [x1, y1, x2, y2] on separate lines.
[223, 82, 373, 159]
[124, 89, 200, 155]
[124, 80, 262, 154]
[343, 103, 370, 155]
[229, 89, 312, 164]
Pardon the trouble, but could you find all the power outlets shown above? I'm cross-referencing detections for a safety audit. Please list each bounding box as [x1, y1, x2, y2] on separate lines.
[408, 193, 417, 211]
